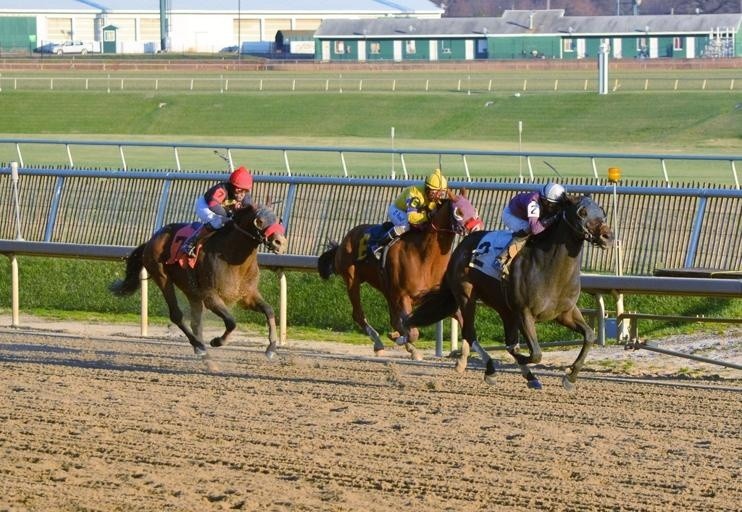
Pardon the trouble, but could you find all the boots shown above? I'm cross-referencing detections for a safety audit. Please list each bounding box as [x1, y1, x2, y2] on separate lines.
[181, 231, 200, 256]
[370, 227, 400, 260]
[493, 237, 521, 271]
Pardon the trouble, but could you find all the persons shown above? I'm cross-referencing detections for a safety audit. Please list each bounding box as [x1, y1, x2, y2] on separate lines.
[491, 182, 570, 276]
[180, 167, 257, 258]
[368, 169, 450, 260]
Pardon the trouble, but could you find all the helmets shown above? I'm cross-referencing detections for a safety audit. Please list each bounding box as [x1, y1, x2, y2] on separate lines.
[540, 183, 565, 203]
[425, 170, 448, 191]
[230, 167, 253, 189]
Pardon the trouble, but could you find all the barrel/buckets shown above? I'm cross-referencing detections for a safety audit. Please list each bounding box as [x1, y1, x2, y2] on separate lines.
[605, 317, 618, 339]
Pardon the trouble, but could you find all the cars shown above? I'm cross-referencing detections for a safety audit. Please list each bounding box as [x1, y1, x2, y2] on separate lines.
[32, 40, 94, 56]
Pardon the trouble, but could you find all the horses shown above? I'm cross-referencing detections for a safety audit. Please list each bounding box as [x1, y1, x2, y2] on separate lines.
[403, 191, 616, 392]
[105, 194, 289, 375]
[316, 185, 485, 373]
